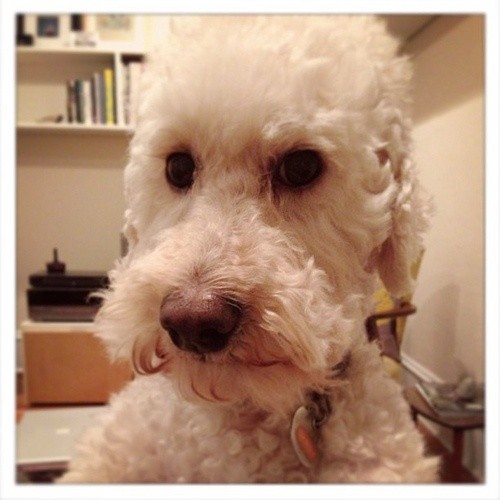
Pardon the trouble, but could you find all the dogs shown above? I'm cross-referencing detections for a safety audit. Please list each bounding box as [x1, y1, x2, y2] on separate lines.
[51, 14, 443, 486]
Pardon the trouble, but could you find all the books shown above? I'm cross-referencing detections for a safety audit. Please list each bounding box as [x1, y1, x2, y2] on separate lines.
[66, 52, 145, 128]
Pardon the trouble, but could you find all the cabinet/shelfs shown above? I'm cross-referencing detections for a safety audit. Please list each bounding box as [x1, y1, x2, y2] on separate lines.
[17, 13, 153, 422]
